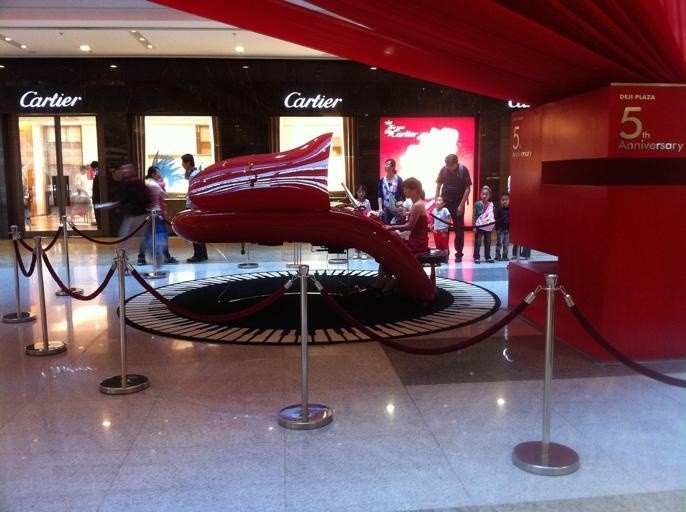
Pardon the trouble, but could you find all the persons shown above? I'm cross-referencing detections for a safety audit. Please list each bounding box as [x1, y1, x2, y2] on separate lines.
[433, 153, 473, 262]
[491, 190, 512, 262]
[135, 166, 180, 266]
[363, 176, 431, 301]
[510, 244, 532, 259]
[375, 158, 408, 231]
[89, 161, 99, 223]
[179, 153, 209, 263]
[73, 165, 98, 226]
[469, 185, 496, 264]
[429, 195, 452, 257]
[347, 183, 373, 261]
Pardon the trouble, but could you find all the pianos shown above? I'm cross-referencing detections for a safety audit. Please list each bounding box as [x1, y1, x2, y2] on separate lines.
[172, 132, 436, 303]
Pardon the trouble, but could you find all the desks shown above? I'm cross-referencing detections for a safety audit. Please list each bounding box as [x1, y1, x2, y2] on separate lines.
[164, 198, 186, 236]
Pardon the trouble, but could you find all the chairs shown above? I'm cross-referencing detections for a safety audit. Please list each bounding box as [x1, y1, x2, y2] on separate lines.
[52, 175, 70, 206]
[65, 196, 92, 223]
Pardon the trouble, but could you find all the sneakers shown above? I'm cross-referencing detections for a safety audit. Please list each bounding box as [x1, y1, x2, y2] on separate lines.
[486, 257, 493, 262]
[474, 258, 480, 263]
[494, 255, 509, 261]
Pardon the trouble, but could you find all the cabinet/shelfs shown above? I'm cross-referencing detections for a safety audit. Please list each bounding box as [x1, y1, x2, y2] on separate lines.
[42, 126, 84, 176]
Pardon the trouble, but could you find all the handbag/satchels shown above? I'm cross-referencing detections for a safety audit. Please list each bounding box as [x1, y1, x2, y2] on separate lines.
[387, 192, 399, 216]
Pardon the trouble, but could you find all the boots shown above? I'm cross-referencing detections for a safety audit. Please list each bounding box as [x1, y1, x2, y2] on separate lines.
[455, 253, 463, 262]
[164, 254, 179, 263]
[137, 255, 146, 265]
[187, 242, 208, 262]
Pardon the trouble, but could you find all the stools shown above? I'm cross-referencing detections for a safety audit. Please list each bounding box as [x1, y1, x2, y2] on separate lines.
[418, 248, 448, 287]
